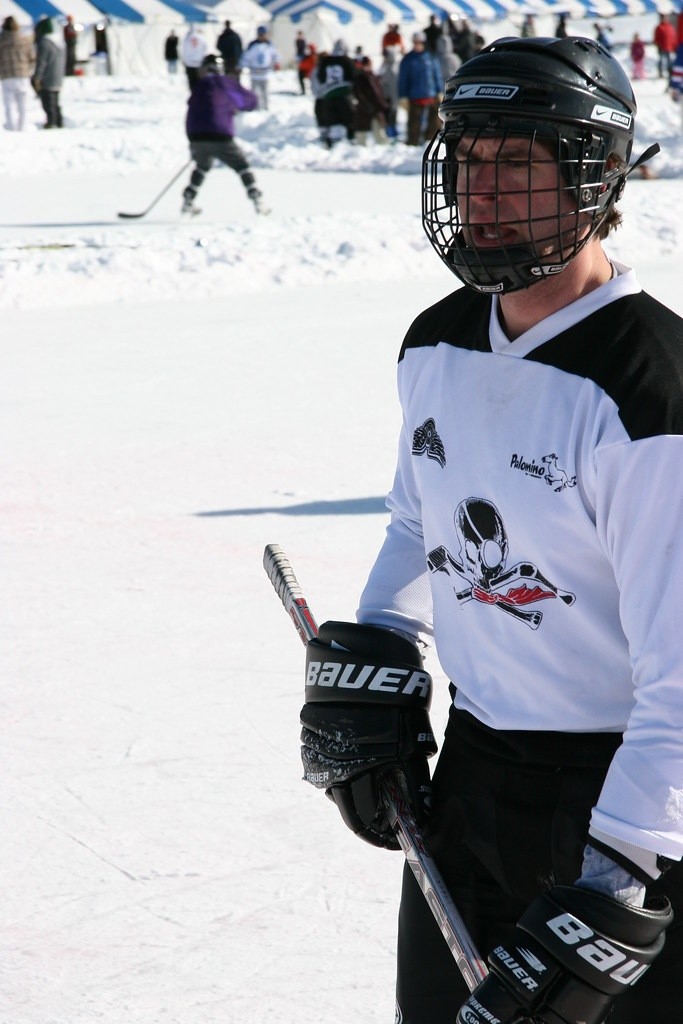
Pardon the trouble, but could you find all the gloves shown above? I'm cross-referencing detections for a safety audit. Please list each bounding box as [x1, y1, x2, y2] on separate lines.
[299, 623, 437, 849]
[451, 870, 674, 1024]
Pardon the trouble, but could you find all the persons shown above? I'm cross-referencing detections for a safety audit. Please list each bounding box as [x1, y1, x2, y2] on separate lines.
[0, 14, 111, 131]
[300, 35, 683, 1024]
[165, 14, 485, 217]
[523, 9, 683, 99]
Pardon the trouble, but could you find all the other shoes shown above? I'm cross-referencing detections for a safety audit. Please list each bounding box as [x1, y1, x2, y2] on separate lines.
[184, 202, 202, 215]
[255, 198, 269, 216]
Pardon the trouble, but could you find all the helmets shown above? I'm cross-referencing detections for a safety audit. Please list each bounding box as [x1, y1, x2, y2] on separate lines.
[200, 55, 226, 76]
[422, 35, 638, 293]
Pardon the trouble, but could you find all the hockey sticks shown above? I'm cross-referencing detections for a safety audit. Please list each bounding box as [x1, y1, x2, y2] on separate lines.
[262, 543, 490, 995]
[118, 156, 193, 219]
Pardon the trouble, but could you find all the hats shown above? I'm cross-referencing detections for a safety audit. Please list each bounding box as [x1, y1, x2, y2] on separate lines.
[34, 17, 54, 34]
[2, 17, 17, 32]
